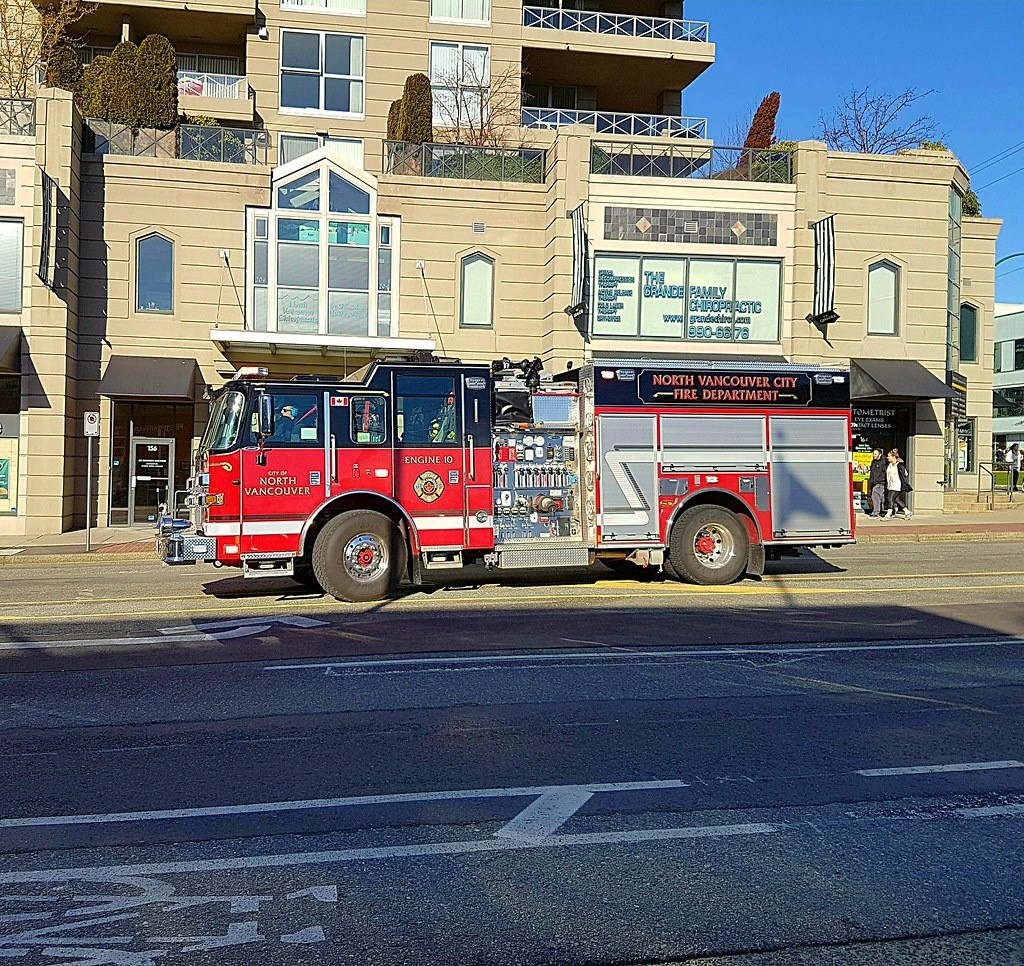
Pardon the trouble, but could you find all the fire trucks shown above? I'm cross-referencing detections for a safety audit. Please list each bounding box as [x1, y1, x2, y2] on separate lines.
[153, 352, 856, 604]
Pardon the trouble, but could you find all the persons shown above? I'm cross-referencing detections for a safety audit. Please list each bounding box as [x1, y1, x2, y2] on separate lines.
[996, 447, 1004, 463]
[867, 447, 889, 519]
[881, 448, 914, 521]
[274, 404, 307, 443]
[1006, 443, 1024, 491]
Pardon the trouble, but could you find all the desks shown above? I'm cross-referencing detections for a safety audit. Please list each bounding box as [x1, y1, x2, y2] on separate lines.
[661, 128, 701, 138]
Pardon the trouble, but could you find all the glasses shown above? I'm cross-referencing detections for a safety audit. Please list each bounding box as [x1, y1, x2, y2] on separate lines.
[282, 409, 291, 412]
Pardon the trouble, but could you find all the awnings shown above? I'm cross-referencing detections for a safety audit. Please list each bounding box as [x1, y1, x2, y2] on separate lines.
[0, 326, 21, 372]
[993, 391, 1016, 407]
[95, 354, 196, 399]
[850, 358, 960, 400]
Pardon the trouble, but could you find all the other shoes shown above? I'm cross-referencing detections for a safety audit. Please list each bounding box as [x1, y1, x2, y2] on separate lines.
[880, 517, 893, 522]
[870, 514, 879, 517]
[1009, 486, 1018, 491]
[890, 515, 896, 518]
[904, 510, 913, 521]
